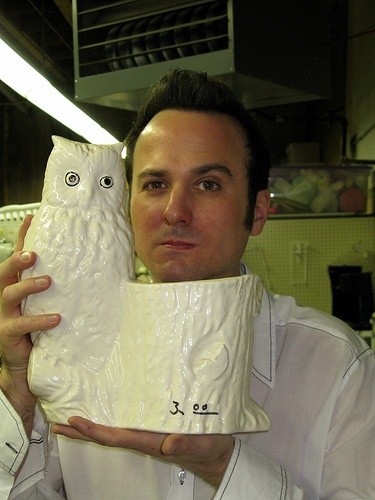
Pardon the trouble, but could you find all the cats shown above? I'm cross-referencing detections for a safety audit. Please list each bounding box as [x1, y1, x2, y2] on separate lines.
[19, 134, 271, 435]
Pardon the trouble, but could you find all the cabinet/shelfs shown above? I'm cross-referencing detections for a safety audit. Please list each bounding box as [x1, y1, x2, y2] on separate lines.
[245, 162, 375, 316]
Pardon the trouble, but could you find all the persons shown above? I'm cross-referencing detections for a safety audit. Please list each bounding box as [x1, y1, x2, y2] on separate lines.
[0, 67, 375, 500]
